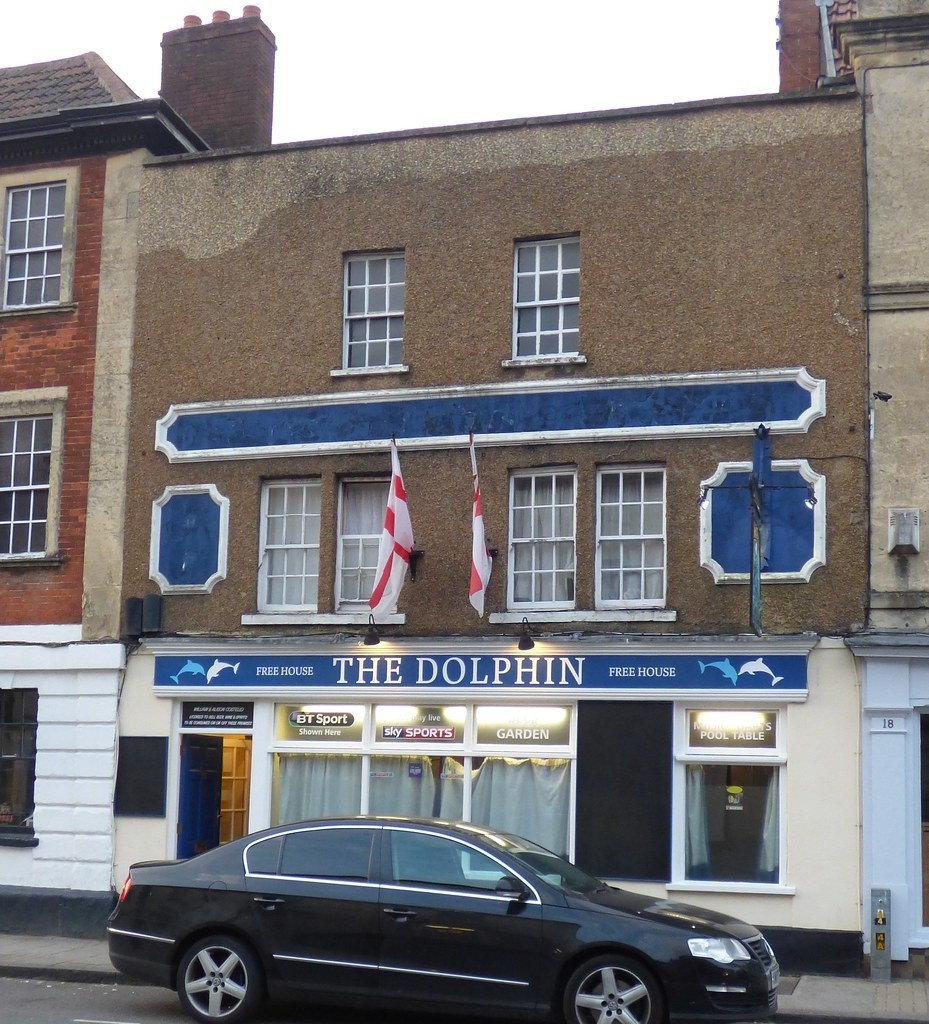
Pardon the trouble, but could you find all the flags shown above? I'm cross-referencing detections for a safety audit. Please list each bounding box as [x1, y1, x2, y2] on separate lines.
[467, 442, 493, 617]
[368, 441, 415, 616]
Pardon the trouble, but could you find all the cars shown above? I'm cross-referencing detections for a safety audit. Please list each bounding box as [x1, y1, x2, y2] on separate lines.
[102, 813, 781, 1024]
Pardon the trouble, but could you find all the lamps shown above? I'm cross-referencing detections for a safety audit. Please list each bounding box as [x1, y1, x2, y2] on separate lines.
[518, 616, 543, 651]
[363, 614, 385, 645]
